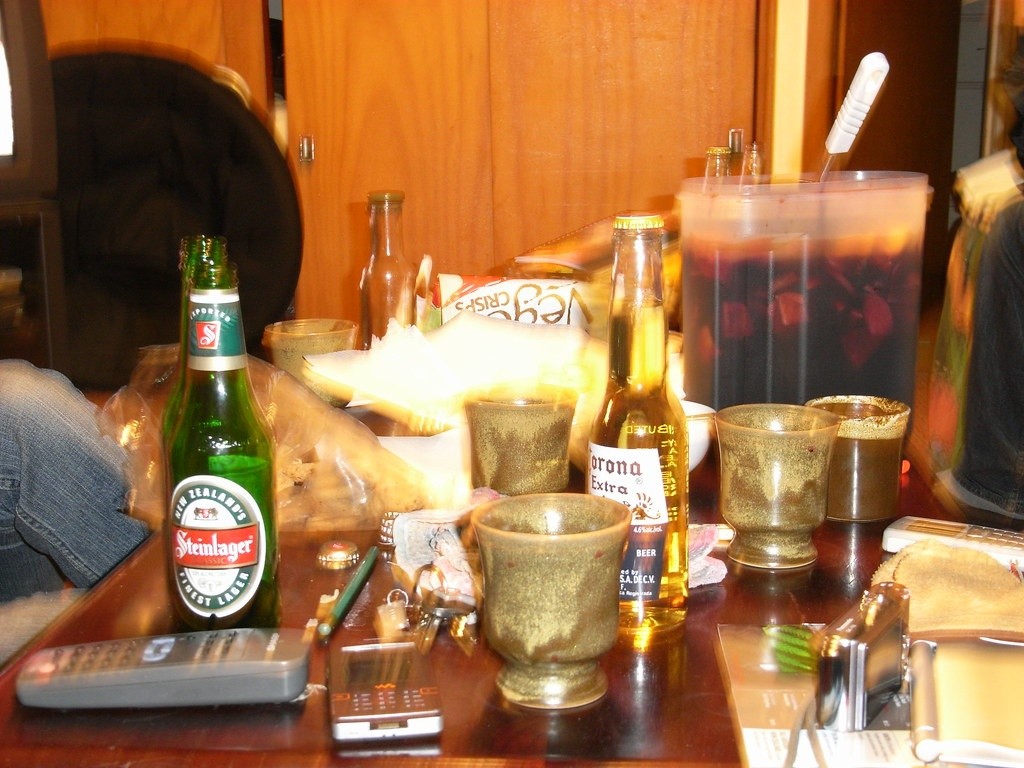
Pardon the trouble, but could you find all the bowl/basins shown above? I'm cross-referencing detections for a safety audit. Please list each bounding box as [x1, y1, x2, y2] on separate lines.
[680, 400, 717, 475]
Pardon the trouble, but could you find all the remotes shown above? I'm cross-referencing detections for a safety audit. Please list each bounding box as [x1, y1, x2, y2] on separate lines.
[881, 516, 1023, 578]
[15, 628, 313, 710]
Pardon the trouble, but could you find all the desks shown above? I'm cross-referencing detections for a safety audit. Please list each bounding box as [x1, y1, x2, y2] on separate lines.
[0, 450, 956, 768]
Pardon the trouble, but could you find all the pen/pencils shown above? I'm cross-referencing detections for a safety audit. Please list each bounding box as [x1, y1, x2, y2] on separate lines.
[317, 545, 379, 636]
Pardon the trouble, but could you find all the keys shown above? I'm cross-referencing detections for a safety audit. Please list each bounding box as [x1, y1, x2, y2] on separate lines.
[375, 565, 482, 657]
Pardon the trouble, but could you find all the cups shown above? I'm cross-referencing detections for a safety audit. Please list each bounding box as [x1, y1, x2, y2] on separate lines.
[471, 491, 634, 709]
[715, 403, 841, 570]
[465, 382, 577, 499]
[806, 394, 910, 523]
[260, 318, 359, 412]
[741, 141, 770, 190]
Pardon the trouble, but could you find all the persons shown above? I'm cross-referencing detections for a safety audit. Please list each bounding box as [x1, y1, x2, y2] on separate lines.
[0, 354, 154, 607]
[950, 200, 1024, 521]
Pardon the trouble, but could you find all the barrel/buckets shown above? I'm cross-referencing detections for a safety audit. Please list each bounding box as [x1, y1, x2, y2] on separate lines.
[680, 171, 935, 414]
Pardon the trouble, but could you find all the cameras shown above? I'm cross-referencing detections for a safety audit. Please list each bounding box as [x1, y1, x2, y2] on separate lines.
[813, 580, 911, 734]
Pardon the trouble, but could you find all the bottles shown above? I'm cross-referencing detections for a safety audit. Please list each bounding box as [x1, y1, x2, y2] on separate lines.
[701, 145, 733, 195]
[357, 190, 418, 357]
[583, 209, 690, 637]
[160, 234, 279, 635]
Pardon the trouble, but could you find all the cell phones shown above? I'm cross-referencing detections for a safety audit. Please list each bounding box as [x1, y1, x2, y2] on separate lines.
[325, 635, 443, 742]
